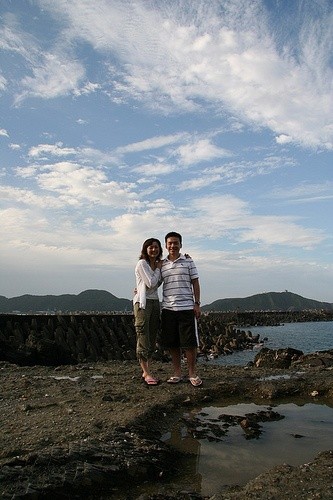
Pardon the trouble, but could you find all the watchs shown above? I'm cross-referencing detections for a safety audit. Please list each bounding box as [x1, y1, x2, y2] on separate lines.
[195, 301, 201, 305]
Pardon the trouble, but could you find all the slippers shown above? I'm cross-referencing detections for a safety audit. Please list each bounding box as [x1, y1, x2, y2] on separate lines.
[189, 376, 203, 387]
[142, 374, 158, 385]
[167, 377, 184, 383]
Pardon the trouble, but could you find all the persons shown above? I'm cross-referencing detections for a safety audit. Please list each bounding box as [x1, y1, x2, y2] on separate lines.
[134, 232, 202, 387]
[133, 238, 192, 385]
